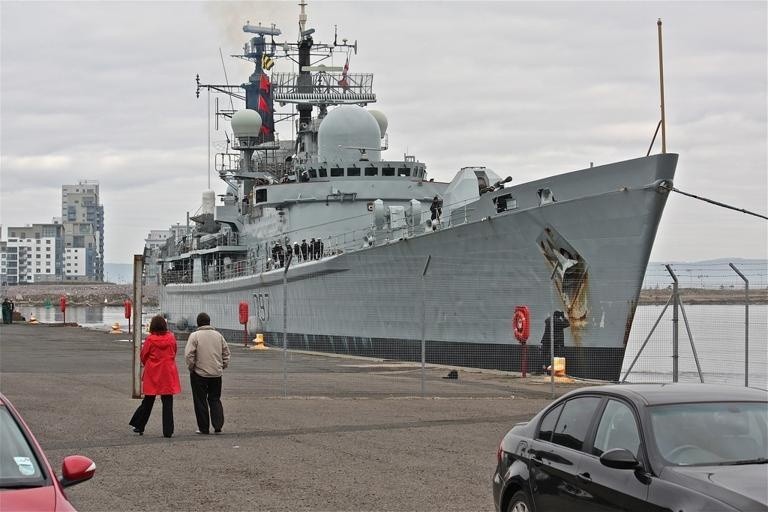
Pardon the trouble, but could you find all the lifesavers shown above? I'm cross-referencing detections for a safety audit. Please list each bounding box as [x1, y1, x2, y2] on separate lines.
[512, 311, 526, 340]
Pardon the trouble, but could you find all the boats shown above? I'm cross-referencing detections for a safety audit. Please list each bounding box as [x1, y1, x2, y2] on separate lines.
[156, 1, 679, 383]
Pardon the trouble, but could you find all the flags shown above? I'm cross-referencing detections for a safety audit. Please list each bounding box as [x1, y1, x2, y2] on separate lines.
[261, 55, 275, 69]
[257, 96, 273, 114]
[259, 124, 273, 138]
[258, 74, 272, 93]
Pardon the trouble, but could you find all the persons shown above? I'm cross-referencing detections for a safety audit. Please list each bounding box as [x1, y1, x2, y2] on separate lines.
[270, 236, 324, 268]
[184, 312, 231, 435]
[1, 298, 14, 325]
[532, 310, 570, 376]
[429, 197, 442, 231]
[127, 315, 182, 437]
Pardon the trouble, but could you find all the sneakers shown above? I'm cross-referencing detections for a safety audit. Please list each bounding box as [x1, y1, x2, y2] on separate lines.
[196, 428, 223, 434]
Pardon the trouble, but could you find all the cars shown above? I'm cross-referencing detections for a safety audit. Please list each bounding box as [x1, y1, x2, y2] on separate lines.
[0, 389, 96, 512]
[492, 382, 768, 512]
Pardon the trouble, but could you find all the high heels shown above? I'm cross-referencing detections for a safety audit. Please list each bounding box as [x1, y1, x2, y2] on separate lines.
[132, 426, 144, 435]
